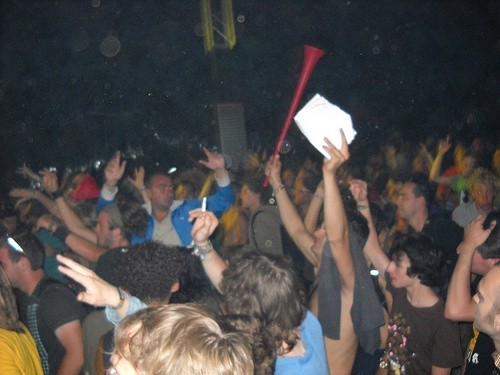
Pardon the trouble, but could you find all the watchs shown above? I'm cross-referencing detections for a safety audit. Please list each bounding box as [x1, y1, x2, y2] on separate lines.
[112, 287, 127, 310]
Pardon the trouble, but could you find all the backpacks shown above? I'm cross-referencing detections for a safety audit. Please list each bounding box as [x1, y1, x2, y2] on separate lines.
[252, 210, 305, 276]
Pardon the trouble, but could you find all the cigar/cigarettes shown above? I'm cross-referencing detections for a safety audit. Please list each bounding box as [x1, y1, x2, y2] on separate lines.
[201, 196, 208, 220]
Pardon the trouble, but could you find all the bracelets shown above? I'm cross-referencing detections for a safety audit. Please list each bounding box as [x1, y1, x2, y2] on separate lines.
[271, 184, 285, 196]
[32, 189, 41, 199]
[49, 189, 63, 202]
[48, 219, 71, 242]
[356, 204, 370, 210]
[313, 192, 325, 202]
[192, 240, 214, 260]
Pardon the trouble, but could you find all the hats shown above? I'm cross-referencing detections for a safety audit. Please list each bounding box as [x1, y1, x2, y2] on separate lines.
[66, 173, 99, 199]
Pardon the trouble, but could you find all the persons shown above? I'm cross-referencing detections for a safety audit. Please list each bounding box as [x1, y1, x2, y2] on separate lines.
[0, 130, 500, 375]
